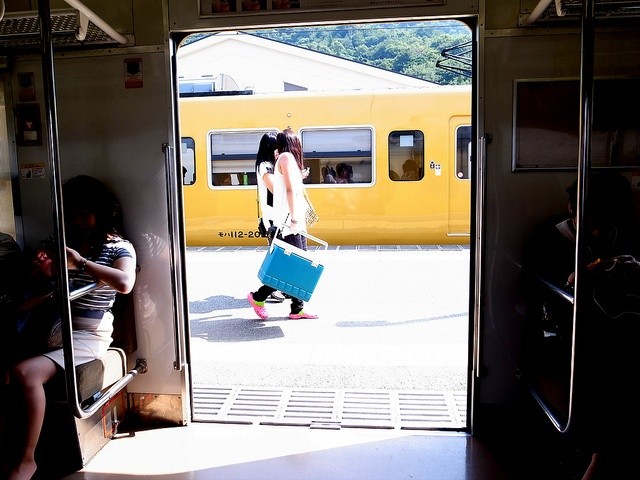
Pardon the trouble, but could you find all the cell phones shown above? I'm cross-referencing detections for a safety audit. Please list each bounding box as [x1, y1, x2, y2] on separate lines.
[39, 240, 57, 255]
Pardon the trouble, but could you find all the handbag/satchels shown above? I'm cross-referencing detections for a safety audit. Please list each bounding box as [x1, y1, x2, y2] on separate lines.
[267, 226, 283, 246]
[258, 217, 266, 237]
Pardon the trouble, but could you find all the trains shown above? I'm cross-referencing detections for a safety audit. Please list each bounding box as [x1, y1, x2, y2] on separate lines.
[179, 84, 471, 248]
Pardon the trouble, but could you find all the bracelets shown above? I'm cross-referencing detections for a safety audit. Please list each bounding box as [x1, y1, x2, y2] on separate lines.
[291, 219, 298, 224]
[564, 280, 574, 292]
[78, 258, 87, 270]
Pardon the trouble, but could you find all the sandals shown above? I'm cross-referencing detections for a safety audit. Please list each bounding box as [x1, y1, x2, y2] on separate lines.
[247, 292, 269, 319]
[289, 310, 318, 319]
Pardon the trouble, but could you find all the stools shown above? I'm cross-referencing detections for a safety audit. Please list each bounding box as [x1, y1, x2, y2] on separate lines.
[536, 374, 582, 429]
[0, 290, 136, 479]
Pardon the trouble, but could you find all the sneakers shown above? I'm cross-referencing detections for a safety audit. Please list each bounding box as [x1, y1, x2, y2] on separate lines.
[271, 290, 285, 301]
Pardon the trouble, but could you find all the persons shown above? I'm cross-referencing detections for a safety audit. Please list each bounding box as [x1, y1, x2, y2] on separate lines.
[248, 128, 320, 320]
[217, 174, 232, 186]
[334, 163, 353, 183]
[515, 169, 640, 480]
[0, 174, 136, 479]
[183, 166, 187, 178]
[255, 132, 310, 301]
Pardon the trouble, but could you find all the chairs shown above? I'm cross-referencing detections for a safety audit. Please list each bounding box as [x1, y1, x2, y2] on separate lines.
[321, 166, 335, 182]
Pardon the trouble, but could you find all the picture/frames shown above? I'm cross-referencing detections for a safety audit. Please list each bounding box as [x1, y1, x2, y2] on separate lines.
[511, 77, 640, 173]
